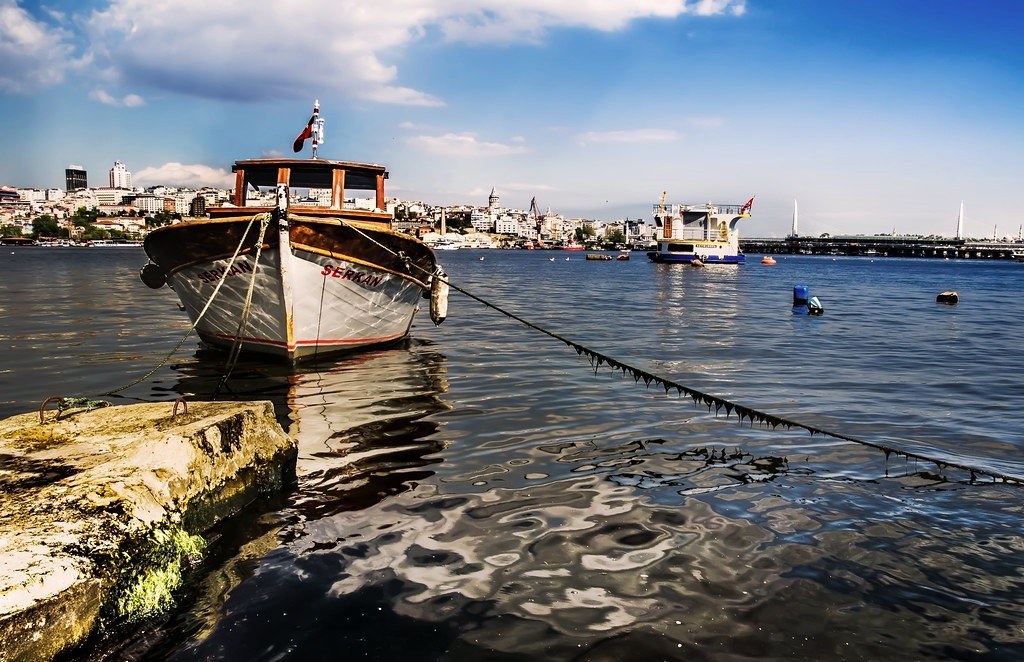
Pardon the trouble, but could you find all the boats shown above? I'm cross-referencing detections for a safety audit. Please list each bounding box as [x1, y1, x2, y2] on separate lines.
[762, 256, 776, 264]
[563, 243, 584, 251]
[586, 254, 612, 260]
[617, 254, 628, 259]
[143, 99, 437, 360]
[646, 191, 756, 263]
[621, 249, 631, 252]
[36, 240, 143, 247]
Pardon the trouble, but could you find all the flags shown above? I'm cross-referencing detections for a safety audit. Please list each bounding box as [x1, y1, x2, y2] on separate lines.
[742, 197, 755, 210]
[294, 115, 314, 151]
